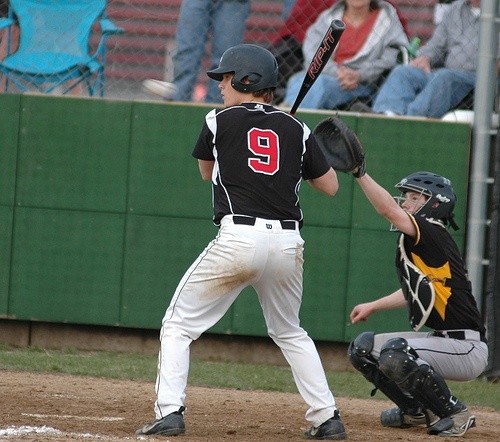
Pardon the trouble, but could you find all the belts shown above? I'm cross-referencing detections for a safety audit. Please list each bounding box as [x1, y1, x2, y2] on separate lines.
[433, 331, 488, 344]
[233, 216, 296, 230]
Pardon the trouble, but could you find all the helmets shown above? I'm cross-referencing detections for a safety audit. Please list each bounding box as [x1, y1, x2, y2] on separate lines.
[389, 173, 457, 231]
[206, 44, 279, 93]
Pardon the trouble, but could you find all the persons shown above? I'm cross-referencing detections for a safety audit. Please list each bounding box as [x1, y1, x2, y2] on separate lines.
[350, 1, 499, 118]
[348, 165, 488, 436]
[135, 45, 348, 440]
[280, 0, 410, 108]
[172, 0, 251, 104]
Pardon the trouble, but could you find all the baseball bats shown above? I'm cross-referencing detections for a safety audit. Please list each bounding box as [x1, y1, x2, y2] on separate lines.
[289, 19, 346, 116]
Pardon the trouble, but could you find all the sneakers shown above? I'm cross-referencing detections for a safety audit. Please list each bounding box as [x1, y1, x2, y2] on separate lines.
[135, 405, 187, 437]
[302, 409, 347, 440]
[380, 403, 441, 428]
[438, 406, 475, 437]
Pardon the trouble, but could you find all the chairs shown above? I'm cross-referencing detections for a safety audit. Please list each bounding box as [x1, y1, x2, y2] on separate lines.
[0, 0, 126, 97]
[434, 2, 500, 131]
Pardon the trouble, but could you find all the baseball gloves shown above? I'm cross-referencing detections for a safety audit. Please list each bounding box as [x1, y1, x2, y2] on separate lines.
[312, 117, 367, 179]
